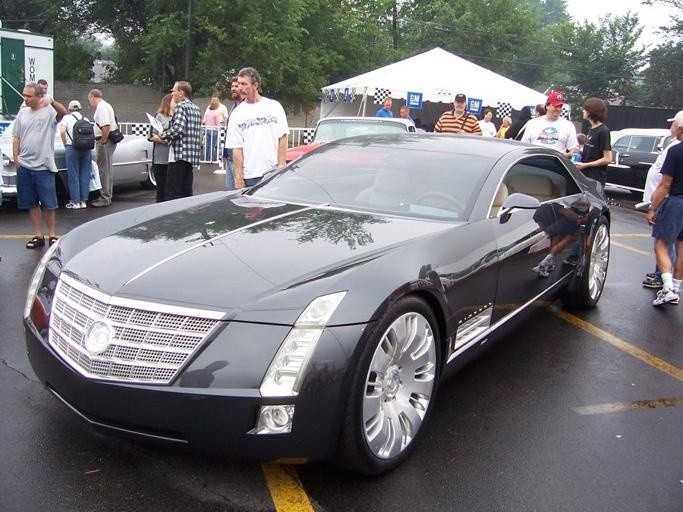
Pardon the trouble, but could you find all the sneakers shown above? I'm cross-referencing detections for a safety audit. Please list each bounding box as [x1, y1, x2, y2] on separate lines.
[563, 257, 577, 267]
[531, 263, 549, 277]
[544, 262, 557, 272]
[66, 200, 112, 209]
[642, 273, 679, 306]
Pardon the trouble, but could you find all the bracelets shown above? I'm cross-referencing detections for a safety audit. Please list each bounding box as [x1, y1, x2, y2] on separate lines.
[647, 205, 658, 212]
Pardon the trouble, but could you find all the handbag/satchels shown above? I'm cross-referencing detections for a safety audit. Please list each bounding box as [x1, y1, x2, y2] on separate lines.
[89, 160, 103, 192]
[109, 129, 125, 144]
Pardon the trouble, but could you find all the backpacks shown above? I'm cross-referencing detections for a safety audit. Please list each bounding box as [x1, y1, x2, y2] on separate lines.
[66, 115, 96, 150]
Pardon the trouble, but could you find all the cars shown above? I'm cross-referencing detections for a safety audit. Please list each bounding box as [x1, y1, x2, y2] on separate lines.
[603, 127, 675, 198]
[281, 115, 423, 168]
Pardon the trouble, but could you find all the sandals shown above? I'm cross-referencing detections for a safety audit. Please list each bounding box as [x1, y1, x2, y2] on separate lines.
[26, 236, 46, 248]
[49, 237, 58, 247]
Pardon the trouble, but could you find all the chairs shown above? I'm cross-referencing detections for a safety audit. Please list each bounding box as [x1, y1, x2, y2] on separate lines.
[487, 177, 508, 220]
[507, 172, 559, 201]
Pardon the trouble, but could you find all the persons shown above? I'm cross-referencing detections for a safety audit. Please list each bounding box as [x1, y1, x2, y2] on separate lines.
[641, 121, 683, 289]
[87, 88, 118, 207]
[373, 84, 613, 199]
[12, 79, 67, 249]
[147, 81, 201, 204]
[646, 109, 683, 308]
[223, 66, 289, 190]
[60, 100, 91, 209]
[202, 97, 228, 166]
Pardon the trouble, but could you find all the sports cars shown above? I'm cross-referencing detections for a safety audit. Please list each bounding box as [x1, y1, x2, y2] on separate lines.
[0, 122, 158, 207]
[15, 130, 612, 480]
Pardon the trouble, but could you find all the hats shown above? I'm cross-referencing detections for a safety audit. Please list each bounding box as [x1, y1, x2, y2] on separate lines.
[455, 94, 466, 101]
[547, 92, 565, 107]
[666, 110, 682, 127]
[69, 100, 82, 110]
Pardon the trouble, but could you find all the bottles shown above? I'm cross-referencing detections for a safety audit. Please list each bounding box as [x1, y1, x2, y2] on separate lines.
[571, 146, 582, 161]
